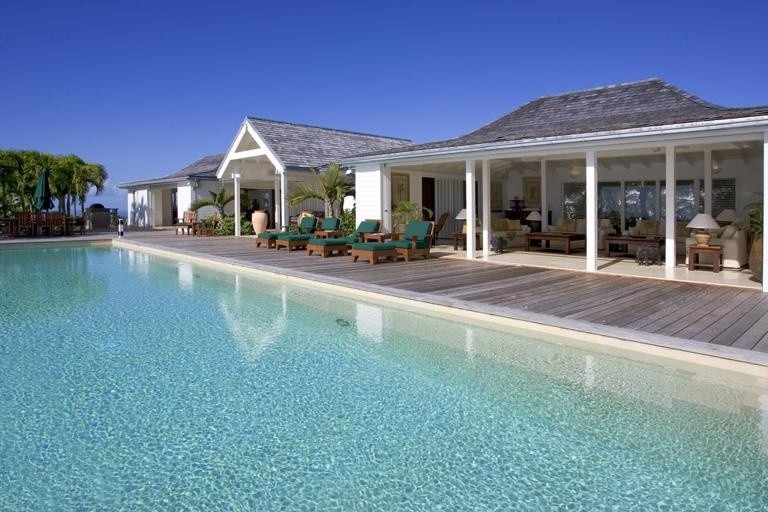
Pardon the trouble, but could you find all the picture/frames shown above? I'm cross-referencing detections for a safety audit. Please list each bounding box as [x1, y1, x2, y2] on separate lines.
[484, 175, 541, 211]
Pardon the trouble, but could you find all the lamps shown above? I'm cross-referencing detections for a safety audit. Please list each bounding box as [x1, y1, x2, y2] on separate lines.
[524, 212, 543, 232]
[455, 209, 467, 233]
[686, 213, 721, 247]
[715, 209, 736, 225]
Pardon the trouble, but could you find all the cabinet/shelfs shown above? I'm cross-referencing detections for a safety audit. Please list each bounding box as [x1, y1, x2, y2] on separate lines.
[504, 198, 553, 248]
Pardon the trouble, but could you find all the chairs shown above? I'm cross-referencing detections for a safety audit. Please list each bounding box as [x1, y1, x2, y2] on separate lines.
[174, 211, 217, 236]
[255, 212, 477, 266]
[8, 210, 66, 236]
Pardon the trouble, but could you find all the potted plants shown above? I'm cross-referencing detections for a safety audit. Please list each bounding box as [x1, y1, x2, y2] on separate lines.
[730, 189, 763, 284]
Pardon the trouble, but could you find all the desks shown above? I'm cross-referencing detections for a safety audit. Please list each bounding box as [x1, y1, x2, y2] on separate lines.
[688, 244, 725, 272]
[522, 230, 666, 262]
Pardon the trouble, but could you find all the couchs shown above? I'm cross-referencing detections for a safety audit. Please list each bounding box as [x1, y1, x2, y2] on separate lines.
[477, 218, 692, 258]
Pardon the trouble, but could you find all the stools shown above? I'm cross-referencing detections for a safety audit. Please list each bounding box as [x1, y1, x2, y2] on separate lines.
[638, 241, 661, 266]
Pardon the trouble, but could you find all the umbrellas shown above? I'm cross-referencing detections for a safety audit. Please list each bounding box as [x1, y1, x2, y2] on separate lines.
[31, 166, 54, 213]
